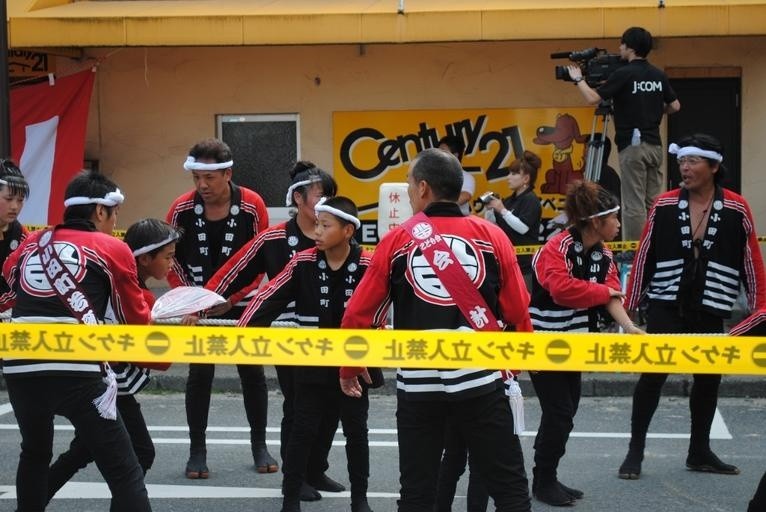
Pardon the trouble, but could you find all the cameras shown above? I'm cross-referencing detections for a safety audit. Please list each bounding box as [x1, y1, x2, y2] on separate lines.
[479, 191, 499, 204]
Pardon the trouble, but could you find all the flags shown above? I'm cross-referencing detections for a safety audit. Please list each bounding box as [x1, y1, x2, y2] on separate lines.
[9, 69, 97, 226]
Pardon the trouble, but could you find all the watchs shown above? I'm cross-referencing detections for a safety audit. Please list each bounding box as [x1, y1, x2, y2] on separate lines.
[573, 76, 586, 86]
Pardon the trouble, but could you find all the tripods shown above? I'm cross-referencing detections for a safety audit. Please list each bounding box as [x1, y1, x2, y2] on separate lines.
[583, 98, 615, 185]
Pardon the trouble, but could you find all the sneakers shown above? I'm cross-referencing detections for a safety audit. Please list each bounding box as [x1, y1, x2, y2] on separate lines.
[185, 450, 209, 479]
[686, 452, 739, 475]
[532, 478, 582, 506]
[252, 445, 278, 473]
[282, 474, 346, 501]
[619, 451, 644, 479]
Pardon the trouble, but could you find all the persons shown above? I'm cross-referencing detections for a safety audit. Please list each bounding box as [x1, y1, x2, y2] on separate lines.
[528, 180, 650, 506]
[164, 138, 279, 479]
[0, 158, 199, 511]
[619, 134, 766, 479]
[729, 307, 766, 511]
[567, 27, 680, 264]
[181, 160, 392, 512]
[339, 135, 542, 511]
